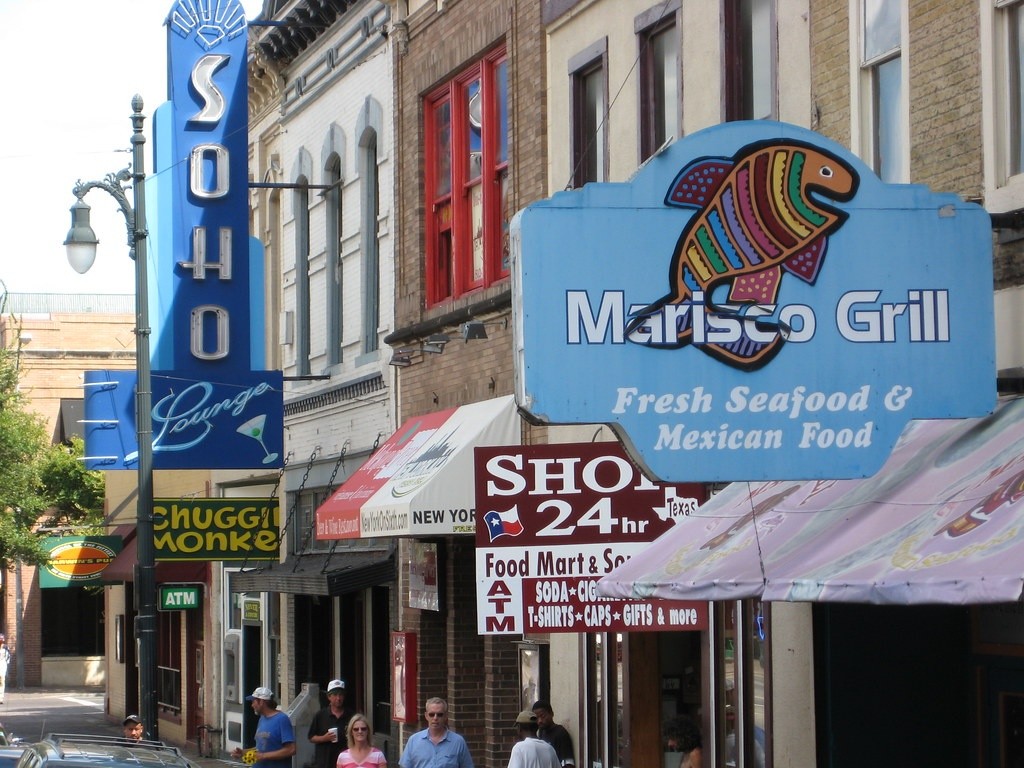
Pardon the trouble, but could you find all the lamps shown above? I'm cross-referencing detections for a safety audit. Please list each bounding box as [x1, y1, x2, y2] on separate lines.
[390, 335, 463, 368]
[463, 315, 507, 345]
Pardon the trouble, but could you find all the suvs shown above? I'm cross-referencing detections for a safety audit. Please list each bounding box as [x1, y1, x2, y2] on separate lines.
[16, 733, 200, 768]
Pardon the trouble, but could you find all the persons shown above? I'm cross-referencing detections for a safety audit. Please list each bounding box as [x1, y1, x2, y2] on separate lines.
[336, 714, 387, 768]
[664, 716, 703, 768]
[111, 715, 156, 750]
[231, 687, 296, 768]
[308, 680, 354, 768]
[508, 712, 562, 768]
[398, 698, 475, 768]
[532, 700, 575, 768]
[0, 635, 11, 704]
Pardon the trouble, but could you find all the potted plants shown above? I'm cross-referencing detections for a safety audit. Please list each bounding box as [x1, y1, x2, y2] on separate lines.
[663, 715, 684, 768]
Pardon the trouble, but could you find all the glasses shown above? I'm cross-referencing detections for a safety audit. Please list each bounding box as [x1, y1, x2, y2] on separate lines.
[353, 728, 367, 731]
[428, 712, 445, 717]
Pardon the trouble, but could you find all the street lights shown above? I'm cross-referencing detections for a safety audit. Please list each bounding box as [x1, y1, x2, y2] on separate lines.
[62, 93, 158, 749]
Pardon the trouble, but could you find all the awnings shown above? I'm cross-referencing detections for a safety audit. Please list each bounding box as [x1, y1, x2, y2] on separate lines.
[596, 391, 1024, 602]
[112, 524, 137, 547]
[317, 393, 522, 540]
[61, 399, 84, 444]
[102, 537, 207, 582]
[231, 550, 398, 595]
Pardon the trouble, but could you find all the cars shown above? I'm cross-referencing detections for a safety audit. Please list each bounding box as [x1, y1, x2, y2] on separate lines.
[0, 746, 26, 768]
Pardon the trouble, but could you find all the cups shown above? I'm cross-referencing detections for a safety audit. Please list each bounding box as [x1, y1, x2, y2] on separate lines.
[328, 728, 338, 743]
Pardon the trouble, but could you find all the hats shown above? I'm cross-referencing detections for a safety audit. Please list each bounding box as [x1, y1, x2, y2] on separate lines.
[326, 679, 346, 694]
[513, 711, 537, 727]
[245, 686, 274, 701]
[123, 715, 142, 725]
